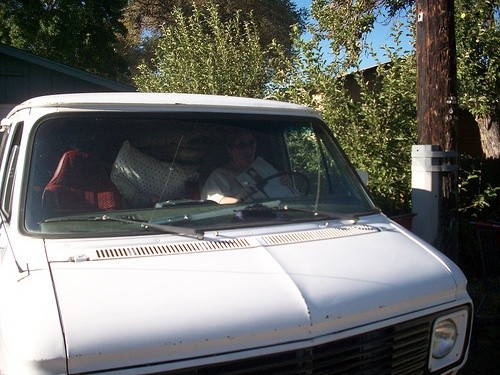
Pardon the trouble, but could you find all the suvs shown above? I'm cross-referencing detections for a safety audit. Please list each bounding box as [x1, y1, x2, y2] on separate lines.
[0, 92, 474, 374]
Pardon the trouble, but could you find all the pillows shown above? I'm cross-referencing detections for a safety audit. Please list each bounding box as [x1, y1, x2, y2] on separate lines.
[110, 140, 200, 208]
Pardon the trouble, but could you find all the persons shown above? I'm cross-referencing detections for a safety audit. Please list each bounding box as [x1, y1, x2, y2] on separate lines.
[201, 124, 302, 204]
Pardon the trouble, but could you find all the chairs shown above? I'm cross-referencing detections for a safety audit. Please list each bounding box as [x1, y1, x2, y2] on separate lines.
[42, 148, 116, 214]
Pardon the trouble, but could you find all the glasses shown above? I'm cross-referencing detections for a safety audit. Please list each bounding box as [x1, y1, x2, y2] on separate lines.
[234, 138, 258, 150]
